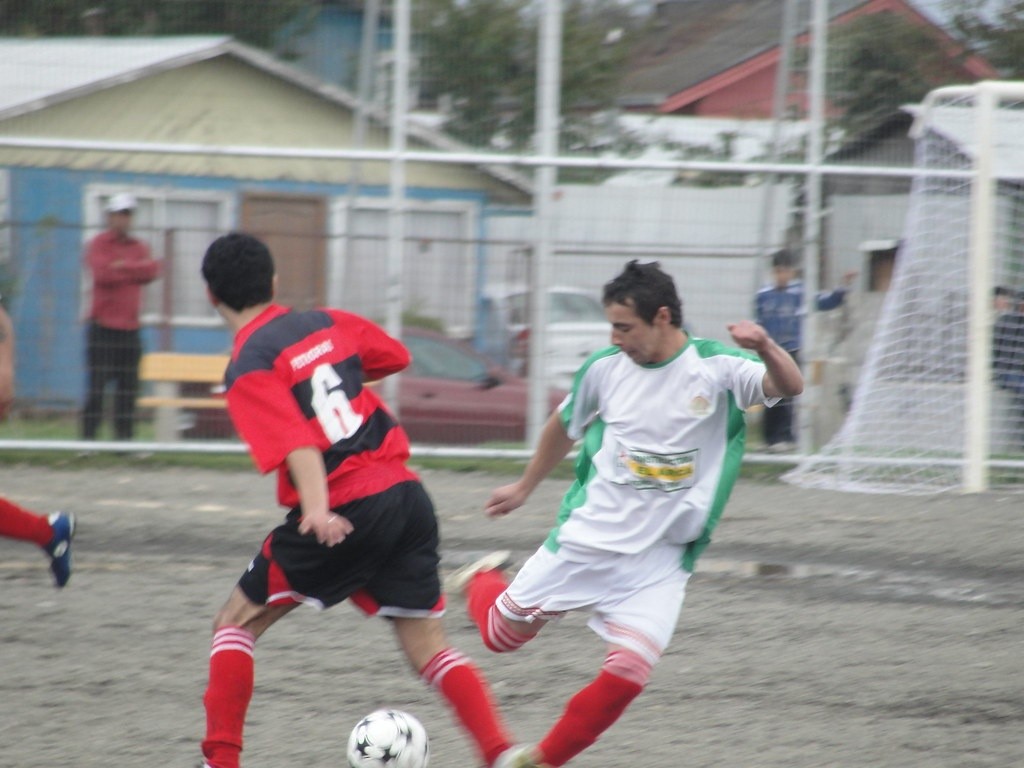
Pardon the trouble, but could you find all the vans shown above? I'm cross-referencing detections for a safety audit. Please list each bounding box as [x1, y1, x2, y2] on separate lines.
[505, 322, 616, 439]
[467, 282, 609, 371]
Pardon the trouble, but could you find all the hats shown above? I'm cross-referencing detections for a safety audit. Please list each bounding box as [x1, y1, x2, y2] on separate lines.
[105, 194, 137, 213]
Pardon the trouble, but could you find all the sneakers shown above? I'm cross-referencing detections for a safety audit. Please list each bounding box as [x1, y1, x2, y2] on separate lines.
[44, 511, 76, 586]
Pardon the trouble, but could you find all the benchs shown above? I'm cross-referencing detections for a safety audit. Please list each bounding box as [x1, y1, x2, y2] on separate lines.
[129, 351, 234, 413]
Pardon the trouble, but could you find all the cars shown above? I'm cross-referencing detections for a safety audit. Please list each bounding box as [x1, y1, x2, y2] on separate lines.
[186, 316, 579, 453]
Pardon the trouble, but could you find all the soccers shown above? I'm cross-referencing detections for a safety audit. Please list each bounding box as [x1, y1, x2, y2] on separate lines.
[348, 708, 429, 768]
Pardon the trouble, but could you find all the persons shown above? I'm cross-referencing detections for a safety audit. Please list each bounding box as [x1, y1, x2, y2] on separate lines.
[987, 286, 1024, 453]
[0, 303, 77, 588]
[450, 258, 804, 768]
[753, 251, 865, 452]
[74, 194, 162, 443]
[193, 233, 534, 768]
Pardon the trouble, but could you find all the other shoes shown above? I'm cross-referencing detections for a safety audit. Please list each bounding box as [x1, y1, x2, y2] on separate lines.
[444, 549, 514, 595]
[492, 743, 550, 767]
